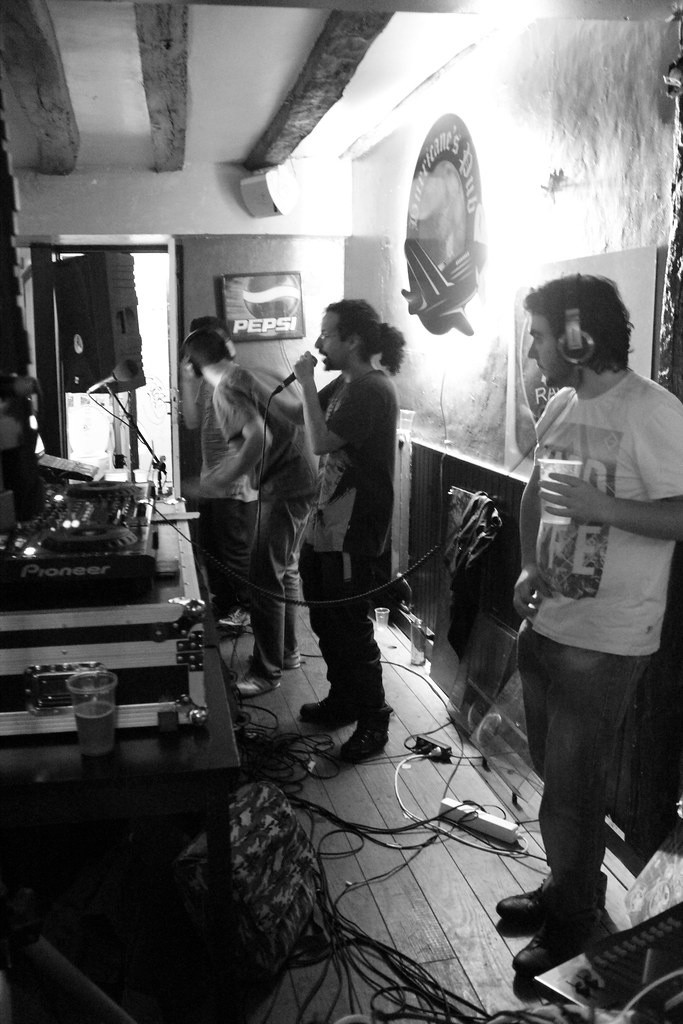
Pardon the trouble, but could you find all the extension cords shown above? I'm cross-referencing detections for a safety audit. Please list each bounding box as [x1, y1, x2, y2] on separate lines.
[417, 734, 451, 760]
[438, 797, 520, 844]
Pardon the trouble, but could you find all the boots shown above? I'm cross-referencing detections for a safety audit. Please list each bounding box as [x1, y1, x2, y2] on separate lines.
[340, 704, 393, 761]
[299, 685, 386, 725]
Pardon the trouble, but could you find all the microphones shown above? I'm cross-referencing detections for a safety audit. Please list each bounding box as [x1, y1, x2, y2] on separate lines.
[87, 359, 138, 393]
[273, 355, 318, 394]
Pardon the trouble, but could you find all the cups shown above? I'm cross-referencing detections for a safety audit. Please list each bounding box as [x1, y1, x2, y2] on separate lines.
[66, 671, 118, 756]
[537, 458, 583, 526]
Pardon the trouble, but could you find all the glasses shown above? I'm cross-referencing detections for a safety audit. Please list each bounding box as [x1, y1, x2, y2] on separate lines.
[320, 332, 337, 339]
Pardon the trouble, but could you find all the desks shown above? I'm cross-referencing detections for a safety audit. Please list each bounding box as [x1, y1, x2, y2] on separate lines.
[0, 504, 238, 1024]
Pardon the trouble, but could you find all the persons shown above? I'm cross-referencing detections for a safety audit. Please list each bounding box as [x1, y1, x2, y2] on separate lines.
[496, 272, 683, 976]
[182, 329, 316, 697]
[292, 299, 406, 759]
[184, 316, 260, 627]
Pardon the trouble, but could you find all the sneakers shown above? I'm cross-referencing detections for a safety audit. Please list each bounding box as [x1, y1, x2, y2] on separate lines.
[496, 872, 609, 927]
[511, 898, 598, 974]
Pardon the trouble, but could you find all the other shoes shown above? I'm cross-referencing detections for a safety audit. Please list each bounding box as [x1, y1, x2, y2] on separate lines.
[283, 652, 301, 668]
[234, 669, 282, 697]
[218, 605, 251, 626]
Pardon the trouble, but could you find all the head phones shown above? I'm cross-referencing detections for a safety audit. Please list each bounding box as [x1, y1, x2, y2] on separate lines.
[557, 273, 596, 366]
[181, 326, 236, 379]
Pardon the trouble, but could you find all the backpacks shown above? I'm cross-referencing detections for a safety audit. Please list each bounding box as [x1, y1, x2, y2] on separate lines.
[172, 781, 324, 979]
